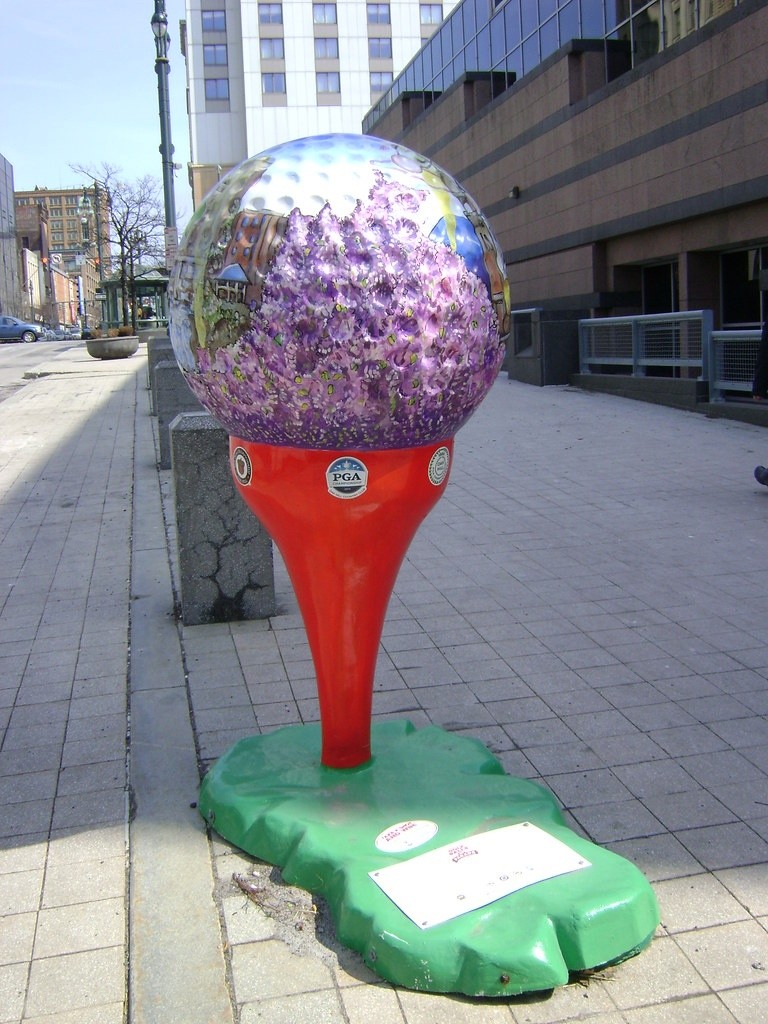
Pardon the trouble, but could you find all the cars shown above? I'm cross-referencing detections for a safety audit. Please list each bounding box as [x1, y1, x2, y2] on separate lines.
[44, 328, 84, 341]
[0, 316, 44, 343]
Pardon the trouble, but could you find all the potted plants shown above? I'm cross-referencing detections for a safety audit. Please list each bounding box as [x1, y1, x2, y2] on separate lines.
[86, 326, 138, 360]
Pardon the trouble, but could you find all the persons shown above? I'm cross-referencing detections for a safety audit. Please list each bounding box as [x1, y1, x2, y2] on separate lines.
[752, 320, 768, 485]
[137, 305, 142, 320]
[146, 304, 153, 319]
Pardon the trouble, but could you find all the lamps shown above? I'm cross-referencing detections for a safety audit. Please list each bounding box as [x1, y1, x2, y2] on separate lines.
[510, 184, 520, 198]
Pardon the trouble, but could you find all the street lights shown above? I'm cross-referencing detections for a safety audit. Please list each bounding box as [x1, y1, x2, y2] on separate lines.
[81, 179, 111, 330]
[150, 0, 179, 275]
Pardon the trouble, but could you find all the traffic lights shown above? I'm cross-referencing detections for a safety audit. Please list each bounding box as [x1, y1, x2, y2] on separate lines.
[95, 257, 102, 273]
[41, 258, 50, 272]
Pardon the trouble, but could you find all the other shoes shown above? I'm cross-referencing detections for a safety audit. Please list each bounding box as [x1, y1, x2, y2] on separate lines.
[754, 466, 768, 486]
